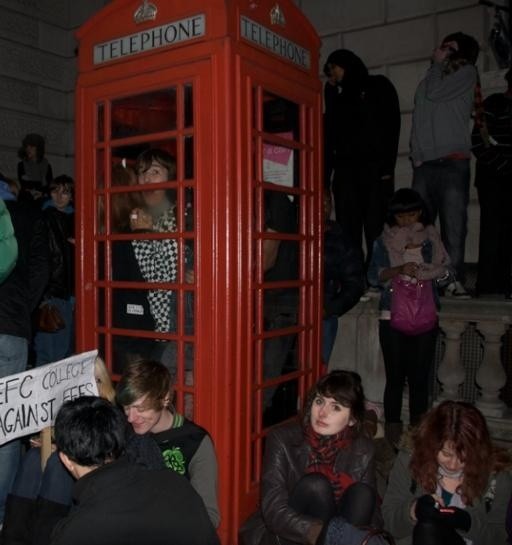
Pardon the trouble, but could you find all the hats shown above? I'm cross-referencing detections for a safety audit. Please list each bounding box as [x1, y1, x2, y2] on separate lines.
[439, 31, 479, 60]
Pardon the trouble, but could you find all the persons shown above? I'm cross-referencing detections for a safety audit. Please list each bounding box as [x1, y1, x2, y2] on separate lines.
[96, 146, 196, 374]
[111, 353, 221, 534]
[0, 357, 120, 544]
[2, 133, 75, 502]
[463, 62, 511, 299]
[264, 48, 455, 455]
[404, 27, 483, 301]
[0, 173, 55, 527]
[378, 397, 512, 544]
[237, 369, 396, 545]
[42, 391, 222, 545]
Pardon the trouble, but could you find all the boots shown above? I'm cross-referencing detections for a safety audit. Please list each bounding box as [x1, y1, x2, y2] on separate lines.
[384, 420, 403, 447]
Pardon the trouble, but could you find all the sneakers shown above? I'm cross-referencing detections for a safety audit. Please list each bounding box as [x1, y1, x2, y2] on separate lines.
[443, 280, 472, 300]
[362, 286, 381, 298]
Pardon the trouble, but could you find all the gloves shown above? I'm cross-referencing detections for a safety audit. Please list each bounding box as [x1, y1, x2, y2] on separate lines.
[414, 494, 471, 534]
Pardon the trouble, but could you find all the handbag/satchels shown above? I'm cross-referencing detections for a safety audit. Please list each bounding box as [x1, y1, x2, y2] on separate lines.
[487, 4, 512, 70]
[36, 303, 65, 332]
[385, 268, 440, 334]
